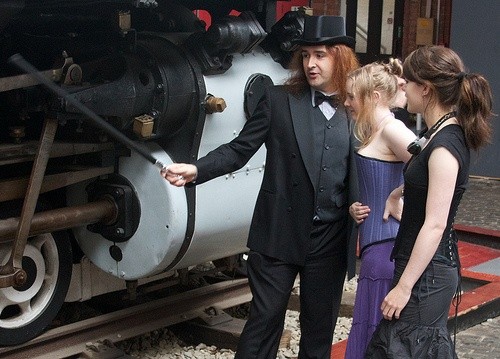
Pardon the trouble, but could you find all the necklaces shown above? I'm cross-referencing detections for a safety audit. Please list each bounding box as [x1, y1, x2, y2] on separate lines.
[362, 44, 497, 359]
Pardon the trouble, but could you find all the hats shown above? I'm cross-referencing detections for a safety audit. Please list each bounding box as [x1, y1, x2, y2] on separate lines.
[291, 14, 356, 48]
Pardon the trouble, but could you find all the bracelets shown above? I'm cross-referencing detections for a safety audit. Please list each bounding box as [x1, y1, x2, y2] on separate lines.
[401, 188, 404, 196]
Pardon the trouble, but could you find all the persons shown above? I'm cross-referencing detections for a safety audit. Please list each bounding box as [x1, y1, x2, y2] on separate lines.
[159, 15, 362, 359]
[344, 56, 417, 359]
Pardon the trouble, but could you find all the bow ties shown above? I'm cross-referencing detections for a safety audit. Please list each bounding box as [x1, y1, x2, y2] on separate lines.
[314, 91, 342, 109]
